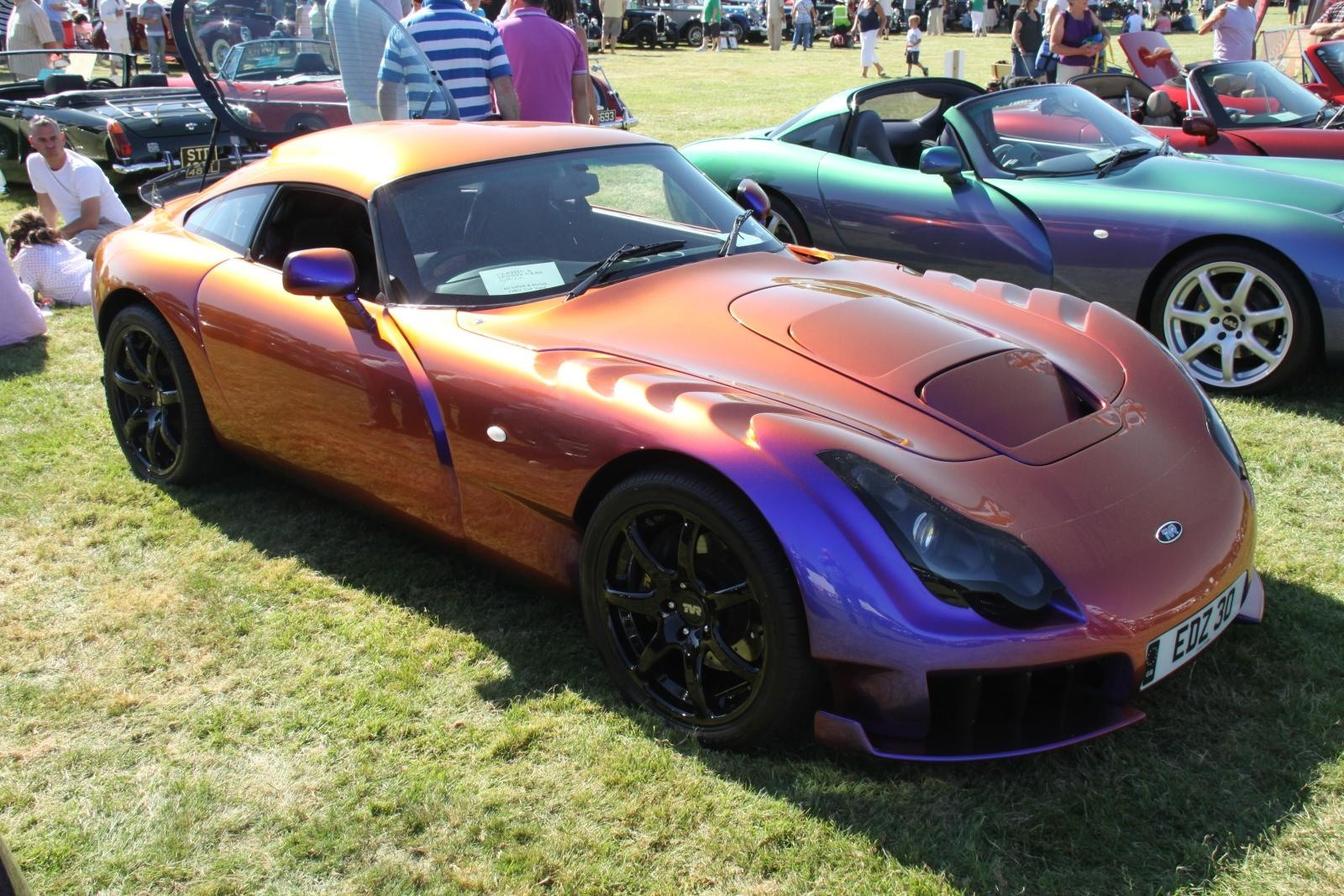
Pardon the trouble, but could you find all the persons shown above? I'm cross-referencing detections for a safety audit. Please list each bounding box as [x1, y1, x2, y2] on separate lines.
[462, 161, 598, 262]
[0, 0, 601, 121]
[889, 0, 906, 34]
[970, 0, 1006, 38]
[0, 235, 47, 343]
[920, 0, 947, 37]
[25, 114, 134, 253]
[599, 0, 626, 53]
[1121, 0, 1214, 38]
[766, 0, 784, 51]
[790, 0, 818, 51]
[830, 0, 886, 76]
[905, 15, 928, 77]
[1284, 0, 1302, 26]
[1198, 0, 1257, 61]
[1009, 0, 1110, 85]
[695, 0, 722, 53]
[8, 208, 92, 306]
[1310, 0, 1344, 40]
[1007, 75, 1039, 88]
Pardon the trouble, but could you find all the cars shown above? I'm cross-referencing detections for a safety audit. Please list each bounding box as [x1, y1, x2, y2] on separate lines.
[576, 0, 835, 52]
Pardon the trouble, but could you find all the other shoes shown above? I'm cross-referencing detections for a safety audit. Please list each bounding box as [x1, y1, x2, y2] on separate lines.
[922, 67, 928, 76]
[904, 73, 910, 76]
[696, 46, 707, 53]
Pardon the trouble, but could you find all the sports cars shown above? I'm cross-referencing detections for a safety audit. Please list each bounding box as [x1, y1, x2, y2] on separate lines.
[490, 65, 640, 130]
[0, 1, 357, 189]
[990, 29, 1344, 160]
[662, 75, 1344, 397]
[90, 120, 1258, 762]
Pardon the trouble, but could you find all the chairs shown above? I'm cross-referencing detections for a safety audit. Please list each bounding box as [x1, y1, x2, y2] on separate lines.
[938, 118, 997, 170]
[294, 52, 327, 75]
[131, 73, 167, 88]
[283, 167, 581, 292]
[1144, 91, 1173, 127]
[847, 109, 899, 166]
[1211, 70, 1256, 98]
[43, 74, 85, 95]
